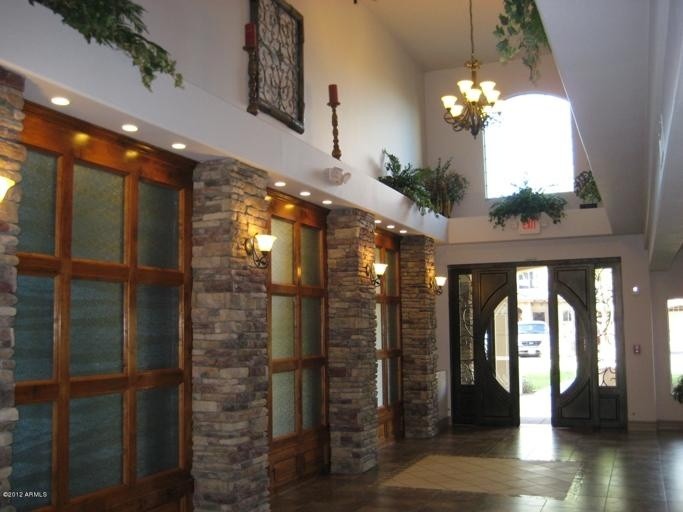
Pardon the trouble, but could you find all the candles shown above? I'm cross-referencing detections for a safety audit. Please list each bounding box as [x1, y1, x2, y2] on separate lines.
[244, 22, 256, 46]
[328, 84, 337, 101]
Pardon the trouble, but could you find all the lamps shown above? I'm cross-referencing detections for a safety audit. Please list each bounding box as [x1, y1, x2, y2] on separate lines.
[364, 262, 389, 288]
[429, 275, 448, 295]
[438, 0, 505, 140]
[244, 232, 277, 269]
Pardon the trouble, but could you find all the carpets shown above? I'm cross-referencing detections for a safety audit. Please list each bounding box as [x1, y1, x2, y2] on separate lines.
[381, 453, 582, 499]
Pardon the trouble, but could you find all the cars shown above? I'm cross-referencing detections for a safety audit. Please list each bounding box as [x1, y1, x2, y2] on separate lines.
[518, 321, 545, 357]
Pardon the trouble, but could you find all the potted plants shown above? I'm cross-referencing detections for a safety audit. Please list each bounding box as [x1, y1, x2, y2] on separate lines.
[423, 156, 470, 218]
[573, 169, 601, 208]
